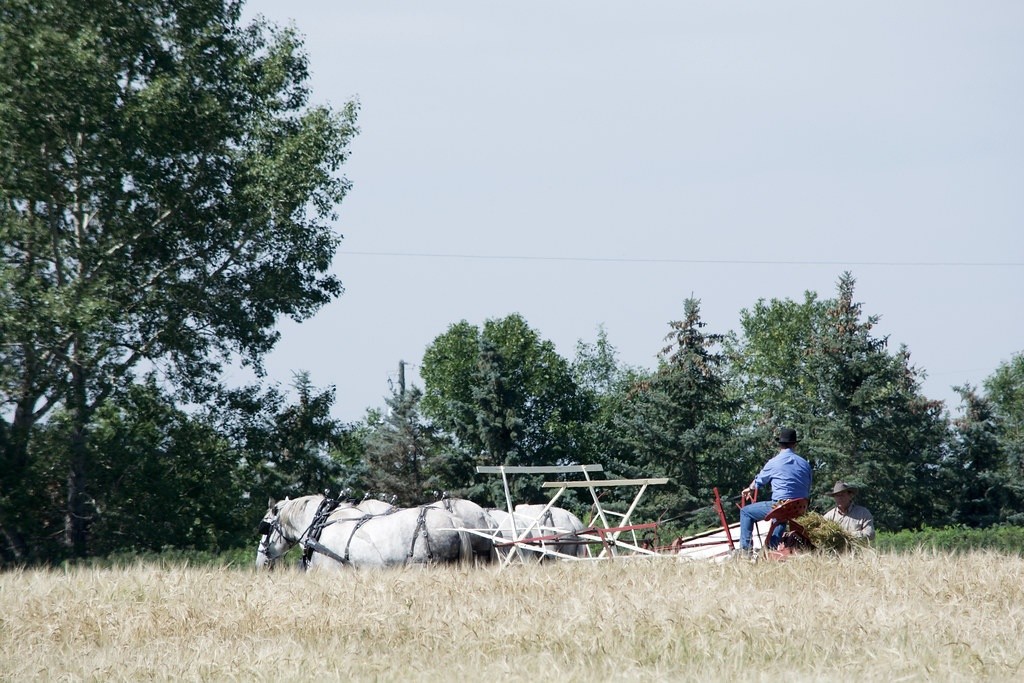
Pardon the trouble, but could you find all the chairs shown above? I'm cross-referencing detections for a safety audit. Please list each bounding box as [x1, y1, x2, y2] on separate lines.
[763, 498, 811, 522]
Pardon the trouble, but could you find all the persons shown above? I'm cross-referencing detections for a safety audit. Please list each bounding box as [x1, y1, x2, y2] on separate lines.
[823, 481, 875, 542]
[739, 429, 813, 560]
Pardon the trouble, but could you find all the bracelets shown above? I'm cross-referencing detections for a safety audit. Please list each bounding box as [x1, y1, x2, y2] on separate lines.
[749, 487, 753, 491]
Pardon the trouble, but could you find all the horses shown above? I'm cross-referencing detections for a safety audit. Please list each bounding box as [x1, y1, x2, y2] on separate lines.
[254, 493, 587, 575]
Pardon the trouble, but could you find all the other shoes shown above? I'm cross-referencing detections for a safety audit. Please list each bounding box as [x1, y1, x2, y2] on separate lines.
[736, 548, 753, 557]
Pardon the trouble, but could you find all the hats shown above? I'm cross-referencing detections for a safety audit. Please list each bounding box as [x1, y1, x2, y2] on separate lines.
[775, 429, 801, 443]
[825, 480, 858, 497]
